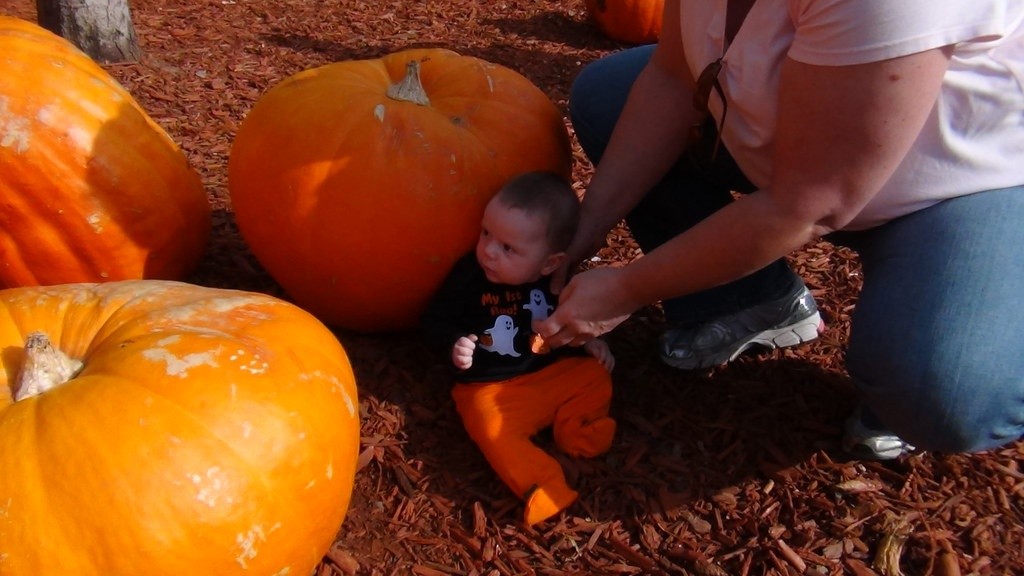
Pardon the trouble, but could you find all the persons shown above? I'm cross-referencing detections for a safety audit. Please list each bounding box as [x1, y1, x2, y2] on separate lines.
[535, 1, 1024, 460]
[417, 171, 617, 524]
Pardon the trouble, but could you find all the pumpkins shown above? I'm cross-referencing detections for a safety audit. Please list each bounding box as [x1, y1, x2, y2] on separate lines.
[0, 280, 362, 576]
[0, 15, 210, 295]
[591, 0, 664, 43]
[231, 51, 573, 340]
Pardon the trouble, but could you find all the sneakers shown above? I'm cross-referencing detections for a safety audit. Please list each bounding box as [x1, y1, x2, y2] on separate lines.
[854, 409, 916, 458]
[659, 287, 825, 368]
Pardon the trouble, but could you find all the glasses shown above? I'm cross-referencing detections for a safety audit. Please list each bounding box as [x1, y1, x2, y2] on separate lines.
[692, 57, 728, 162]
